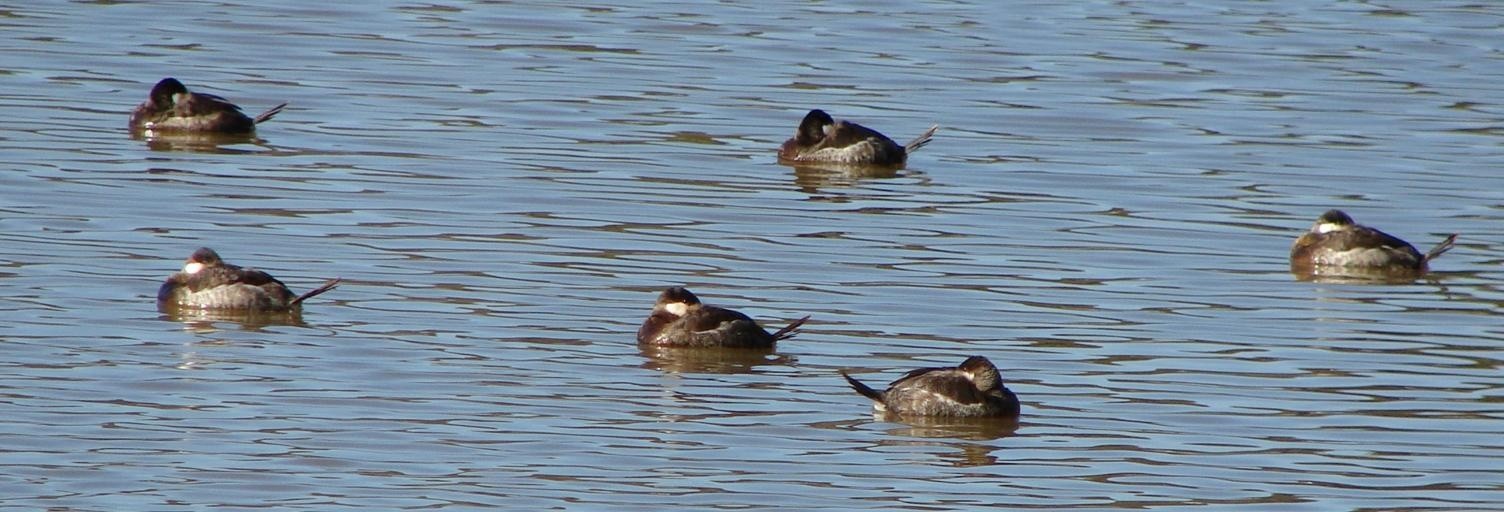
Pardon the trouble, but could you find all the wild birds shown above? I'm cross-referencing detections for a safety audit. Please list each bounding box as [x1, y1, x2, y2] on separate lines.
[128, 77, 289, 140]
[158, 246, 341, 316]
[777, 109, 939, 163]
[636, 285, 811, 348]
[838, 355, 1021, 417]
[1290, 209, 1459, 268]
